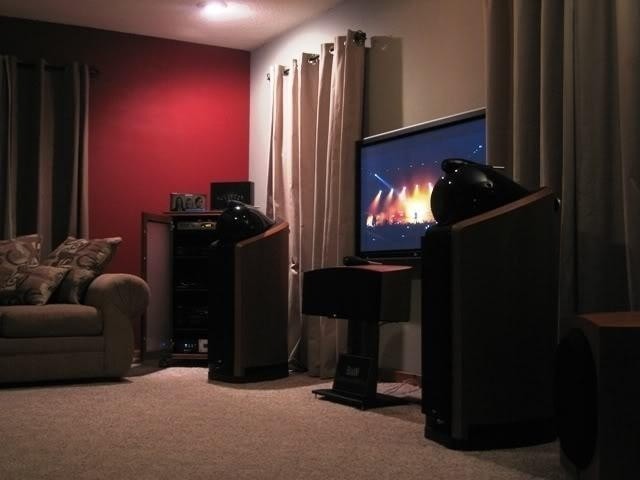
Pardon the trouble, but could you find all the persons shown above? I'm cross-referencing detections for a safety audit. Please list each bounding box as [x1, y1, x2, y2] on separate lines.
[173, 196, 185, 211]
[195, 197, 203, 207]
[185, 197, 193, 208]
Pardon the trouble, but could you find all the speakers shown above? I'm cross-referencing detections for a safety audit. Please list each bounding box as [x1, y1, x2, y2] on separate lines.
[302, 266, 412, 322]
[420, 157, 565, 452]
[211, 182, 253, 209]
[208, 199, 290, 383]
[558, 311, 640, 480]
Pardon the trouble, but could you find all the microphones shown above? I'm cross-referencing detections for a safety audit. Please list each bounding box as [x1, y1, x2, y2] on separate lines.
[343, 256, 384, 265]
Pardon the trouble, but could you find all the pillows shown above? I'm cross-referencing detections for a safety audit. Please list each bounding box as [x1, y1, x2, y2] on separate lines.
[0, 234, 122, 307]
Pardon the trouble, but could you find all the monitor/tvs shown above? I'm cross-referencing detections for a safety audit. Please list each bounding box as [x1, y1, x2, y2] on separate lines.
[354, 109, 486, 256]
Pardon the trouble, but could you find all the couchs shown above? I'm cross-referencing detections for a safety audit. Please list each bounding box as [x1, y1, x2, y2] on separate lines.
[0, 273, 151, 384]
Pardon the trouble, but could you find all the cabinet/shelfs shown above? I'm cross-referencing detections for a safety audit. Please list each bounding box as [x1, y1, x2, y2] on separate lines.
[141, 210, 224, 368]
